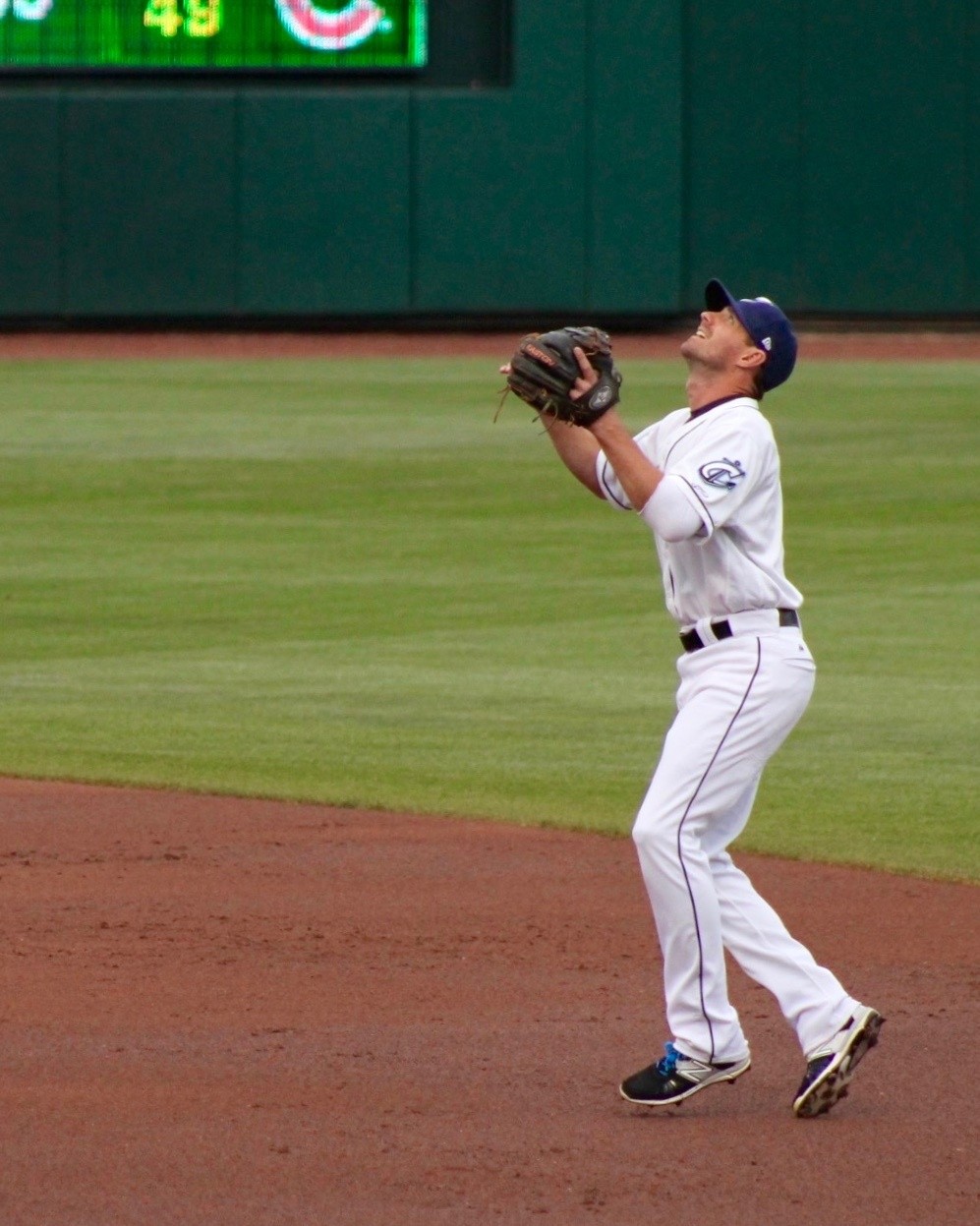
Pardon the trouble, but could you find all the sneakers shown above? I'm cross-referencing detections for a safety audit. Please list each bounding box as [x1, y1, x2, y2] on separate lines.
[618, 1041, 751, 1107]
[791, 1003, 885, 1118]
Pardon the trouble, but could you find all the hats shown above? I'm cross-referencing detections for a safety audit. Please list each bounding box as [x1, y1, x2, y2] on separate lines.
[705, 276, 798, 385]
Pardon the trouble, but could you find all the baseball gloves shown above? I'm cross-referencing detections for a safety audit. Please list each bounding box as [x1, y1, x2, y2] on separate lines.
[492, 323, 625, 438]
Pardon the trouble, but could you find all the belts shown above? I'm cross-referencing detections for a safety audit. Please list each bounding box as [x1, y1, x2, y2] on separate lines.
[678, 608, 799, 652]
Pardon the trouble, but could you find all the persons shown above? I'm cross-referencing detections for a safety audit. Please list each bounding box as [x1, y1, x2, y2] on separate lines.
[500, 277, 885, 1118]
